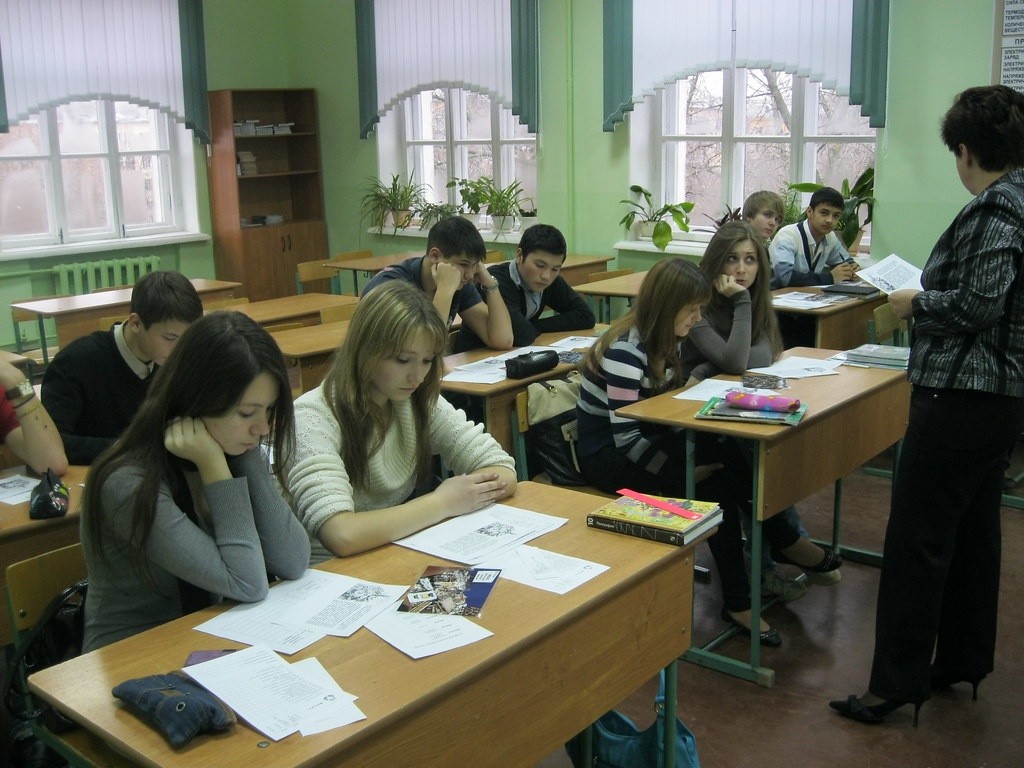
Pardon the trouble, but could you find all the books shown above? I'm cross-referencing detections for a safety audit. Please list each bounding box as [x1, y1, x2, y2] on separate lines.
[847, 343, 911, 370]
[821, 284, 879, 299]
[585, 494, 724, 546]
[232, 118, 294, 136]
[240, 214, 283, 227]
[236, 151, 257, 175]
[693, 397, 808, 426]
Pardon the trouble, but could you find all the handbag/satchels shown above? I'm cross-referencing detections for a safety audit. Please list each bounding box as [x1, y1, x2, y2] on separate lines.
[526, 370, 589, 487]
[564, 668, 701, 768]
[6, 580, 88, 734]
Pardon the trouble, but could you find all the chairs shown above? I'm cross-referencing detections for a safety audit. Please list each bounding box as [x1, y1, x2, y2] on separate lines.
[867, 301, 910, 349]
[292, 257, 340, 299]
[5, 542, 135, 768]
[507, 387, 659, 502]
[585, 268, 637, 325]
[9, 298, 58, 385]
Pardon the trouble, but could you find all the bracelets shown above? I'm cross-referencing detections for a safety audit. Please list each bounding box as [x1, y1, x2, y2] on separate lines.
[12, 393, 36, 409]
[16, 403, 41, 421]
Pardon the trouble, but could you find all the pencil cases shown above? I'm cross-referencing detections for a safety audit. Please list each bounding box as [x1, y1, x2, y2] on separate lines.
[29, 467, 70, 519]
[111, 671, 238, 749]
[743, 371, 786, 389]
[726, 391, 801, 413]
[505, 350, 560, 379]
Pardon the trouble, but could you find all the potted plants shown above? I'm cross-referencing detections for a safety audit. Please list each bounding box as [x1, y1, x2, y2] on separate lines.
[356, 167, 435, 241]
[791, 168, 876, 257]
[398, 199, 465, 234]
[448, 176, 485, 231]
[617, 184, 694, 249]
[469, 177, 531, 248]
[515, 207, 536, 232]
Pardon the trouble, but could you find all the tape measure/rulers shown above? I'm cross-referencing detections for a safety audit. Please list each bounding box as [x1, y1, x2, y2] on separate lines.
[616, 487, 701, 521]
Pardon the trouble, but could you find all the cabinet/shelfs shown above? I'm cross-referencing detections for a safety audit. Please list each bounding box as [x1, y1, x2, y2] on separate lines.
[207, 87, 332, 301]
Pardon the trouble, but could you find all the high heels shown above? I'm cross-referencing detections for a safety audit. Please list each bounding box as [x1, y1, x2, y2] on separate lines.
[929, 663, 986, 701]
[829, 695, 928, 727]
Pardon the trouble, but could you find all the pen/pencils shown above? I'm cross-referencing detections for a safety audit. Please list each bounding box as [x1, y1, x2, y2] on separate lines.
[839, 252, 845, 261]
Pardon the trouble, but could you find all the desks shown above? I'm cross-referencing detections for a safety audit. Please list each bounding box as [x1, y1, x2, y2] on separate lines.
[2, 251, 913, 767]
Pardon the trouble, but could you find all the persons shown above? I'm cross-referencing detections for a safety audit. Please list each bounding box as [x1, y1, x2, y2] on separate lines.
[275, 278, 517, 570]
[825, 85, 1024, 728]
[361, 216, 514, 412]
[578, 257, 842, 648]
[0, 357, 68, 479]
[768, 187, 862, 288]
[455, 224, 595, 354]
[743, 191, 785, 261]
[42, 271, 203, 465]
[81, 309, 311, 661]
[676, 221, 841, 605]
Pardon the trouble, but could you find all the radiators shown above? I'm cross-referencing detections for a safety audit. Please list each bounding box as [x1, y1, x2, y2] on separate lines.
[51, 254, 161, 297]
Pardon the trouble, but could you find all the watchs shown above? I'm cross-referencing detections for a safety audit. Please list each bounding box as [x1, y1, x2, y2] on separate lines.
[4, 379, 34, 400]
[481, 276, 499, 293]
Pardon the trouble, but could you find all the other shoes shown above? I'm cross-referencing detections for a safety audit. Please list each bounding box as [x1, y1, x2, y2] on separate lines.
[1002, 476, 1017, 489]
[769, 540, 844, 572]
[721, 605, 782, 646]
